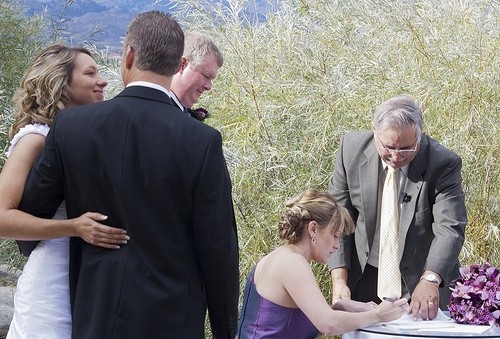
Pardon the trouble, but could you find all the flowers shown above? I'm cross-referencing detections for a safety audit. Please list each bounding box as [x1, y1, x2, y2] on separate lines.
[187, 106, 214, 121]
[445, 263, 500, 329]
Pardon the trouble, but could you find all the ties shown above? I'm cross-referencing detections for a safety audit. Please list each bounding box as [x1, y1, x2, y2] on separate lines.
[376, 164, 402, 302]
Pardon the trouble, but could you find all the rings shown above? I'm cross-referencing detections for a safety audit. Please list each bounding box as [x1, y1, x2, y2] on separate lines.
[429, 301, 435, 305]
[401, 305, 404, 310]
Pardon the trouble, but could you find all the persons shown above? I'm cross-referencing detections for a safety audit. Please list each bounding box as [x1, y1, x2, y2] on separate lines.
[15, 42, 241, 339]
[327, 97, 469, 320]
[0, 42, 131, 339]
[169, 34, 224, 122]
[234, 188, 410, 339]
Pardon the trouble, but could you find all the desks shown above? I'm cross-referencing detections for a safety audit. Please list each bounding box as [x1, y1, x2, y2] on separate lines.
[341, 310, 500, 339]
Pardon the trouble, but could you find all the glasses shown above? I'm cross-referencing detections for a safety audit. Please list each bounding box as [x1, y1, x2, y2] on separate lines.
[376, 132, 420, 156]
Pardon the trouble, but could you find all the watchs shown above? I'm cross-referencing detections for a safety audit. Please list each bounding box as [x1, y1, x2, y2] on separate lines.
[420, 273, 440, 285]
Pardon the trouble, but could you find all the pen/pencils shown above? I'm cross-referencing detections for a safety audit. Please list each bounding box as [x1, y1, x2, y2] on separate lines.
[383, 297, 396, 303]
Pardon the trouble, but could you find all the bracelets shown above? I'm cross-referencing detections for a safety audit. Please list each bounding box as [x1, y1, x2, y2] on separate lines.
[376, 308, 383, 322]
